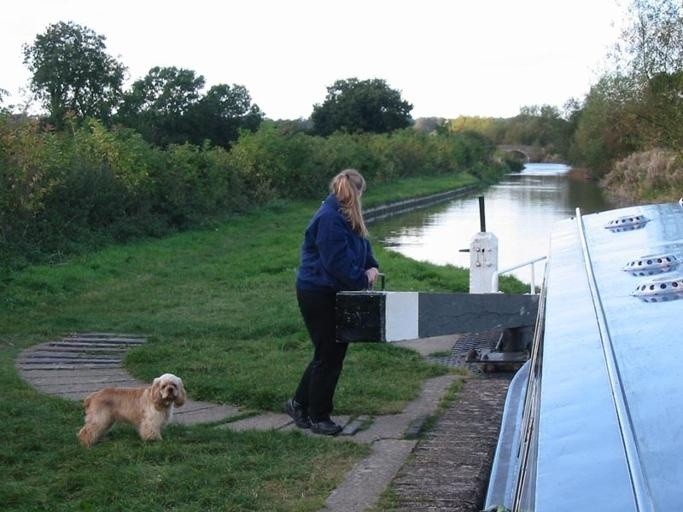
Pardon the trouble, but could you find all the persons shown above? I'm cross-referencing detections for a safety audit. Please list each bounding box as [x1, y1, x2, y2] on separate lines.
[285, 168, 379, 435]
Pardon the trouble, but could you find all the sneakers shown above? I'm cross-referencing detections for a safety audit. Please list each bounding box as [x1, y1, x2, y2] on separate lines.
[282, 397, 312, 431]
[307, 415, 344, 436]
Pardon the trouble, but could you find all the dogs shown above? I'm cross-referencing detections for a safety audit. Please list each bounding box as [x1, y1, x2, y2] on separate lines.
[77, 373, 186, 447]
